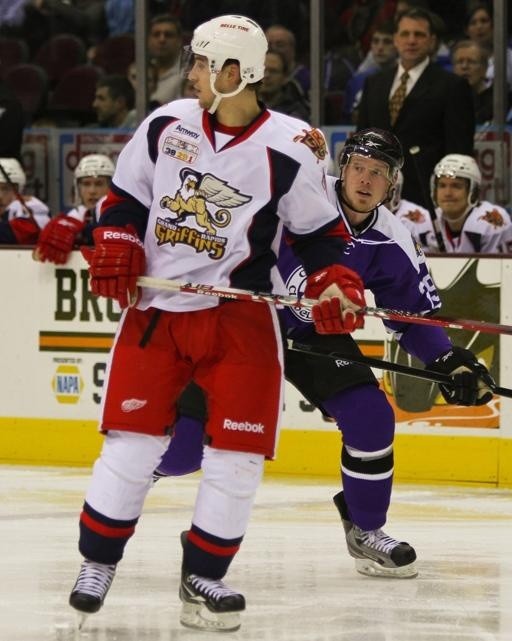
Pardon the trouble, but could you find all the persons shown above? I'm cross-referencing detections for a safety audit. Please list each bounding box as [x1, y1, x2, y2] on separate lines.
[0, 1, 512, 265]
[70, 13, 365, 612]
[153, 128, 497, 569]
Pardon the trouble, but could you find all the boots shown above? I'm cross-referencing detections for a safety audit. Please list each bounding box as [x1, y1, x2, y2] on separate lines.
[333, 490, 416, 568]
[69, 558, 116, 612]
[179, 530, 245, 612]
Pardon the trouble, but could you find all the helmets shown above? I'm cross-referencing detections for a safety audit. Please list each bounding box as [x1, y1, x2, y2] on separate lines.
[430, 152, 482, 194]
[339, 126, 406, 185]
[73, 153, 115, 186]
[0, 156, 26, 193]
[190, 13, 269, 83]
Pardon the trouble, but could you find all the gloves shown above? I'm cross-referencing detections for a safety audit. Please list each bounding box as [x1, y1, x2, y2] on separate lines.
[305, 263, 367, 335]
[31, 213, 83, 264]
[87, 223, 146, 308]
[434, 346, 497, 406]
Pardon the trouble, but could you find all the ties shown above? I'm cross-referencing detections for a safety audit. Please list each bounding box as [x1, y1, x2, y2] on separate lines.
[388, 70, 409, 127]
